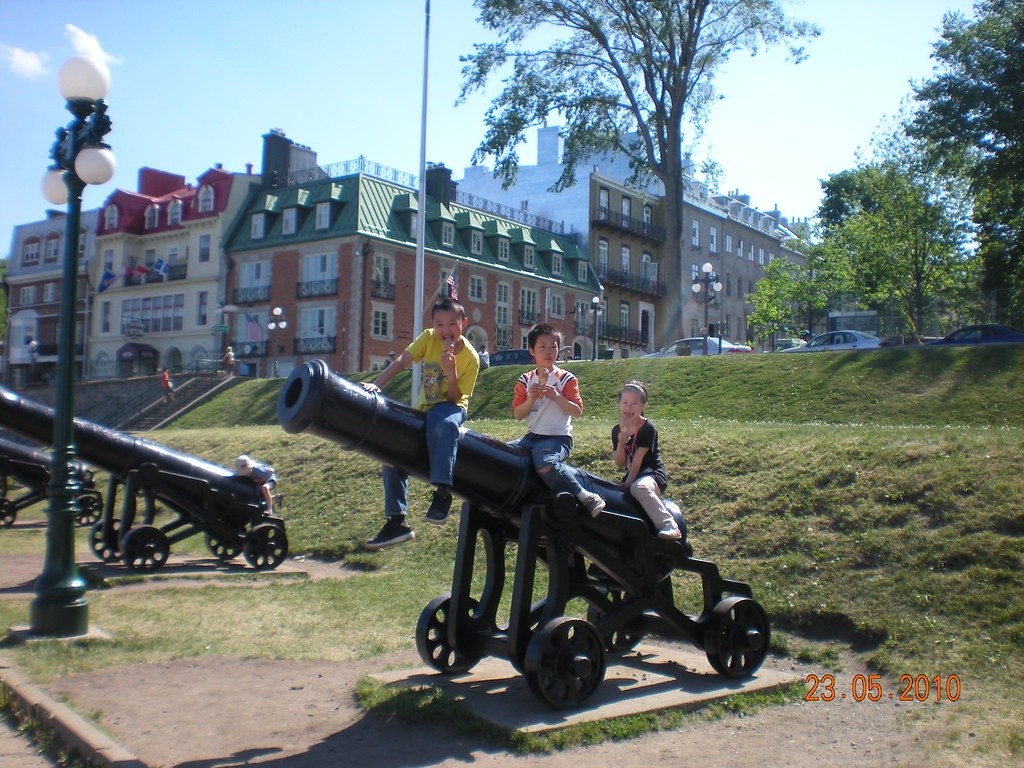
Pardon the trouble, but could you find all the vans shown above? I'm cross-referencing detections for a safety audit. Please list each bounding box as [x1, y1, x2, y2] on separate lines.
[489, 349, 537, 367]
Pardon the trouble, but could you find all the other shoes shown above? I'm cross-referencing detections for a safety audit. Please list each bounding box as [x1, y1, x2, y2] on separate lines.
[659, 526, 682, 541]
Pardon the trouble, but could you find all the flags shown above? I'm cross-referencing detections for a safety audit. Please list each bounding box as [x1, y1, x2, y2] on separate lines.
[446, 263, 457, 302]
[98, 259, 172, 293]
[245, 312, 263, 341]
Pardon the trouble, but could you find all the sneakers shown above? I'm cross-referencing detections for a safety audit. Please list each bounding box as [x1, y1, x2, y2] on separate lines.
[584, 491, 605, 518]
[424, 488, 453, 525]
[366, 518, 416, 551]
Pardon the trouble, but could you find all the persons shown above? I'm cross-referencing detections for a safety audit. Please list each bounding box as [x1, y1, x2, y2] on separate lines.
[161, 368, 174, 403]
[476, 345, 490, 372]
[222, 346, 234, 378]
[506, 321, 606, 517]
[359, 296, 479, 548]
[611, 382, 682, 539]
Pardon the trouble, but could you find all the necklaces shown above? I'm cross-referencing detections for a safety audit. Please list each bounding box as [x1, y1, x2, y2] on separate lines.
[622, 416, 642, 456]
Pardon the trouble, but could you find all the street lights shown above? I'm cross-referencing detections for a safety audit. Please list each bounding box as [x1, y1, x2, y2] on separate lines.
[588, 296, 604, 361]
[716, 320, 727, 354]
[268, 307, 287, 378]
[692, 263, 723, 355]
[768, 317, 778, 352]
[29, 340, 39, 387]
[29, 56, 117, 640]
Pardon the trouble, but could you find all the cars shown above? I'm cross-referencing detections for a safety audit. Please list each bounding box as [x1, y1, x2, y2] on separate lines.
[779, 330, 891, 353]
[640, 336, 753, 358]
[919, 324, 1024, 346]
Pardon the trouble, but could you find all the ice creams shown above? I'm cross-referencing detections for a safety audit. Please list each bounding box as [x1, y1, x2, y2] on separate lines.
[444, 338, 454, 360]
[538, 368, 550, 386]
[618, 414, 627, 433]
